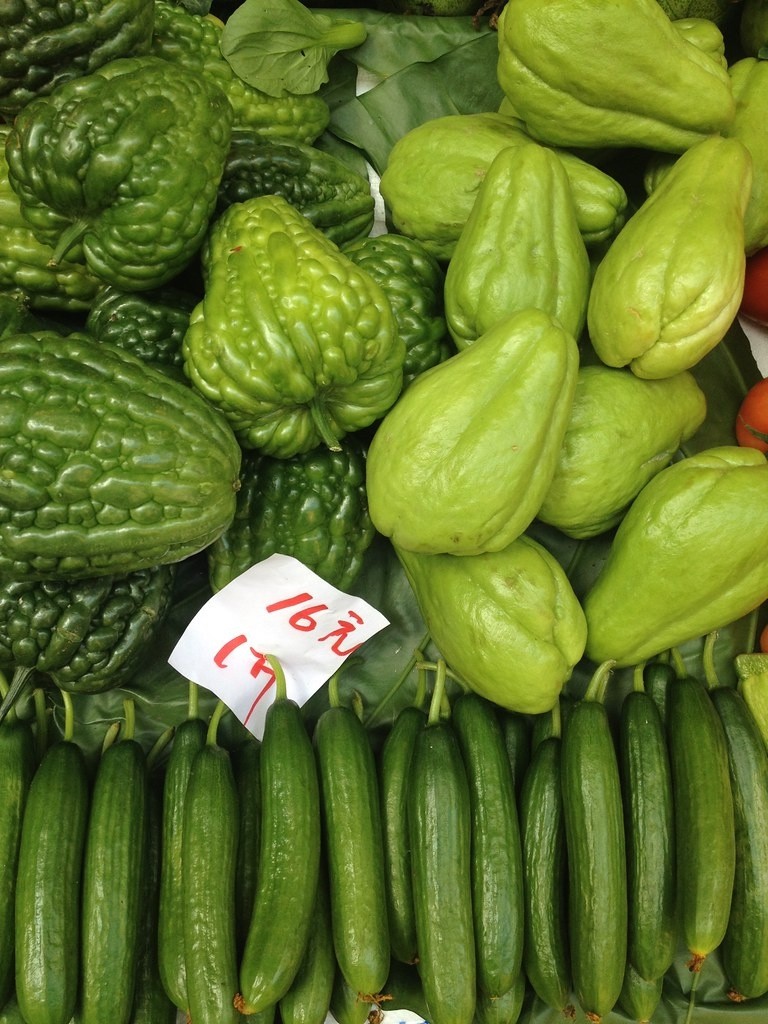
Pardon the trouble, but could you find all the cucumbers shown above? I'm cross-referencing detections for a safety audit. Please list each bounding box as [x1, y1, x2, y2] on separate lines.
[0, 618, 768, 1024]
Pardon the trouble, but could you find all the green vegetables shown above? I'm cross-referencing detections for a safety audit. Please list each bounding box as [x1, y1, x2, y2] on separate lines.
[219, 0, 368, 97]
